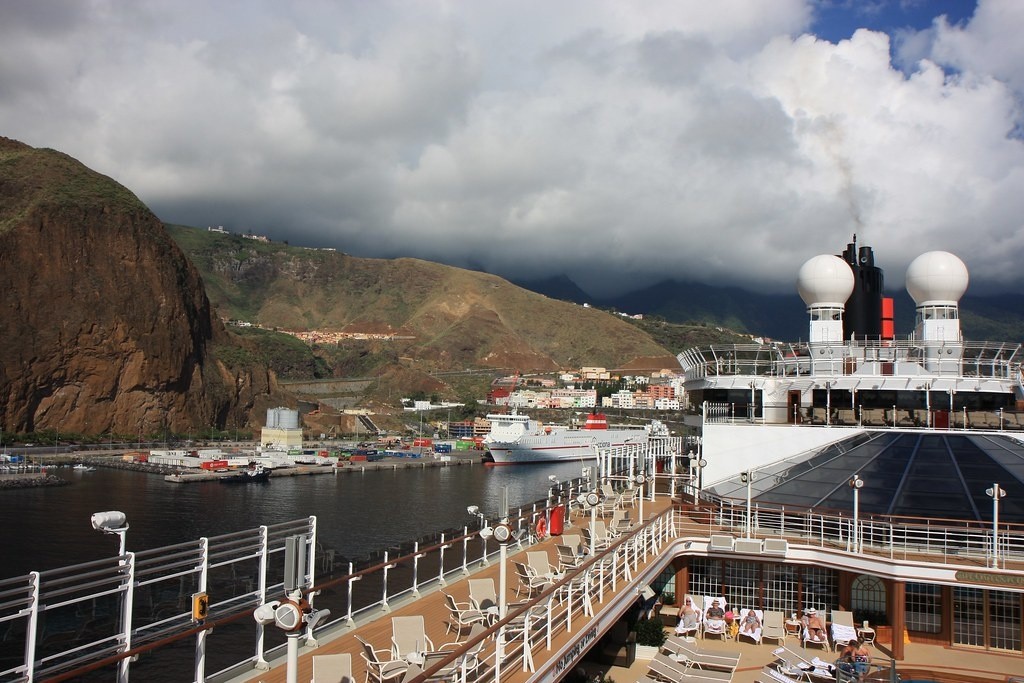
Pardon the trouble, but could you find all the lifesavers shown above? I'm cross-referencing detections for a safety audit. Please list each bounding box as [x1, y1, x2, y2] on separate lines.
[536, 518, 547, 538]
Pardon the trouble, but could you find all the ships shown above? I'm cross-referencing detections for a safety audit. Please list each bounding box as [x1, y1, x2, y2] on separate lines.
[480, 411, 650, 464]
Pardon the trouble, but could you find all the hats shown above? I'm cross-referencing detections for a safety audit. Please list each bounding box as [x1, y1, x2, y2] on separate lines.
[711, 598, 721, 605]
[807, 607, 817, 615]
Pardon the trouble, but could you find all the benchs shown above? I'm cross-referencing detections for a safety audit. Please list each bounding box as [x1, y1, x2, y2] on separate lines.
[672, 492, 716, 524]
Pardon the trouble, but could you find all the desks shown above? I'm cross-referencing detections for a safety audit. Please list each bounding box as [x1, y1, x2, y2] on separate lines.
[669, 654, 687, 663]
[731, 615, 741, 625]
[679, 636, 697, 646]
[784, 620, 803, 640]
[487, 606, 499, 641]
[544, 572, 565, 605]
[407, 650, 428, 665]
[857, 627, 876, 648]
[780, 666, 802, 680]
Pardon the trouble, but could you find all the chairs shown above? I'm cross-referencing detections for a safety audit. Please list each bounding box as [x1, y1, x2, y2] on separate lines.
[702, 596, 727, 642]
[736, 608, 764, 645]
[831, 609, 856, 653]
[802, 608, 829, 653]
[310, 484, 659, 683]
[674, 594, 703, 637]
[635, 634, 741, 683]
[755, 645, 836, 683]
[761, 610, 786, 646]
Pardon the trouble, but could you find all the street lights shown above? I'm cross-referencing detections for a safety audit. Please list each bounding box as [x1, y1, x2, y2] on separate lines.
[741, 468, 756, 540]
[248, 533, 331, 682]
[849, 474, 865, 553]
[986, 484, 1007, 569]
[478, 517, 508, 664]
[689, 445, 707, 512]
[626, 470, 654, 550]
[577, 490, 603, 596]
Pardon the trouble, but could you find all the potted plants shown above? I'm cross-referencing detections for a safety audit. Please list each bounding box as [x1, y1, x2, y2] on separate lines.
[635, 614, 668, 661]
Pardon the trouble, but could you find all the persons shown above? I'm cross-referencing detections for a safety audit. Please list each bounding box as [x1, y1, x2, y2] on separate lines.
[840, 636, 871, 663]
[802, 608, 825, 642]
[741, 610, 759, 633]
[678, 597, 700, 637]
[706, 599, 725, 630]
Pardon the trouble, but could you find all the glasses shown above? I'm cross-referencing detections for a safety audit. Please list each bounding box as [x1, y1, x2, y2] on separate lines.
[686, 600, 691, 603]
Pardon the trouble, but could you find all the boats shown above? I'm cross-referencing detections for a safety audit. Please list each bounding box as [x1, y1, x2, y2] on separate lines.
[0, 464, 103, 474]
[235, 460, 275, 486]
